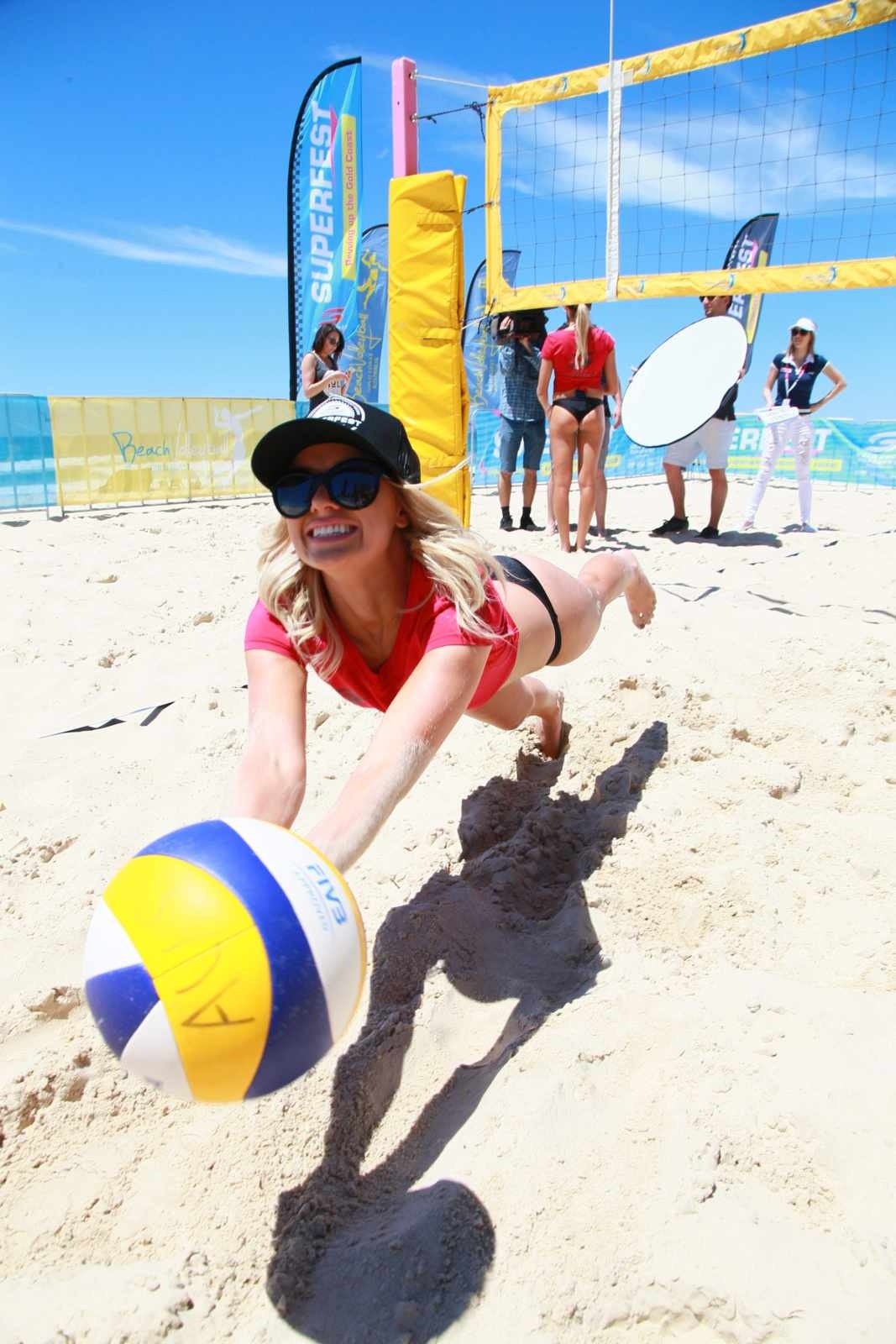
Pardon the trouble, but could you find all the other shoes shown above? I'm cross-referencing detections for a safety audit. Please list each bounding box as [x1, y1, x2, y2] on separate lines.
[519, 517, 546, 531]
[694, 527, 719, 539]
[652, 516, 689, 536]
[500, 515, 513, 531]
[738, 523, 753, 531]
[801, 524, 817, 533]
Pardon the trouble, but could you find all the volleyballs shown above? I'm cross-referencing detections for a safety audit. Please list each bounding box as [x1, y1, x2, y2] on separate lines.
[80, 817, 368, 1104]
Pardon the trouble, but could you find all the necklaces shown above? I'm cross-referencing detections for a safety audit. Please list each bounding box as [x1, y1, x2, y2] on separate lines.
[343, 601, 388, 663]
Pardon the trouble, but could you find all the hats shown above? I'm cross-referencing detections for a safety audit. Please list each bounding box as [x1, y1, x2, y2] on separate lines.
[251, 393, 421, 494]
[789, 318, 817, 332]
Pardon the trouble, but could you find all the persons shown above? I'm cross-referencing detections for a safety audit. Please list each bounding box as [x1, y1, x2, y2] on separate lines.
[534, 302, 624, 554]
[490, 313, 547, 535]
[206, 397, 657, 876]
[303, 323, 352, 415]
[740, 316, 848, 536]
[649, 296, 743, 543]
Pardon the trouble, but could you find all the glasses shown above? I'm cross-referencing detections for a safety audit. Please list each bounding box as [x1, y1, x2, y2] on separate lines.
[699, 296, 717, 303]
[268, 457, 398, 519]
[791, 329, 809, 336]
[329, 336, 340, 347]
